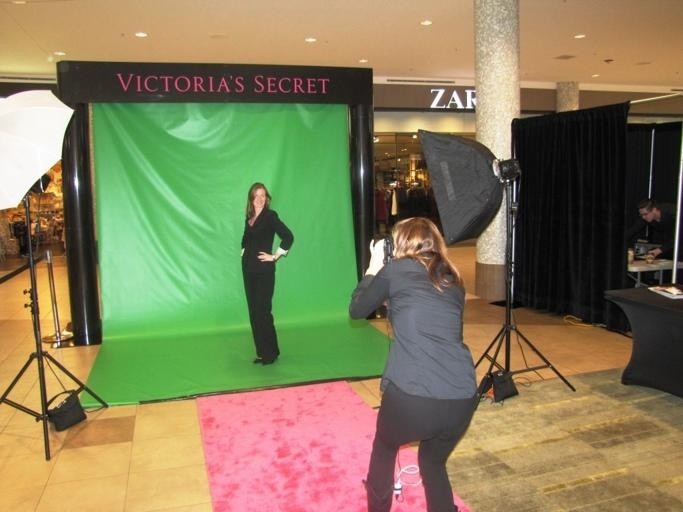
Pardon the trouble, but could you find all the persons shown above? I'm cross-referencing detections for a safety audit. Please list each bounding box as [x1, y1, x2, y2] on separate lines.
[624, 197, 683, 264]
[238, 181, 294, 367]
[348, 216, 477, 512]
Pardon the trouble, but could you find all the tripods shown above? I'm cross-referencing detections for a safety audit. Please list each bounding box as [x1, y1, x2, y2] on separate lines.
[0, 196, 109, 460]
[474, 181, 576, 411]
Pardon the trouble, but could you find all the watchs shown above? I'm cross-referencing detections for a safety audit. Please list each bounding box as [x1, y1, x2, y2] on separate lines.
[271, 253, 277, 263]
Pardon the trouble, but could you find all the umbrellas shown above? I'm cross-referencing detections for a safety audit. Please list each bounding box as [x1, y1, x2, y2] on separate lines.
[0, 83, 78, 212]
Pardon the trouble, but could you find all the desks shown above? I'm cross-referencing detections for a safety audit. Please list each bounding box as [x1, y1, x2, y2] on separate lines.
[604, 256, 682, 396]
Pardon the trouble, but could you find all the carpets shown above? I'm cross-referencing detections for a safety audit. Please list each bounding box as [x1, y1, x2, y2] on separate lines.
[195, 380, 467, 510]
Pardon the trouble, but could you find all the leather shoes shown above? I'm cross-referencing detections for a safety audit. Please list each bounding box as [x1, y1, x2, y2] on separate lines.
[253, 354, 278, 365]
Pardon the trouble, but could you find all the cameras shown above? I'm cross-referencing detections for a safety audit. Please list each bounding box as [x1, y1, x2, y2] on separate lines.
[373, 233, 393, 263]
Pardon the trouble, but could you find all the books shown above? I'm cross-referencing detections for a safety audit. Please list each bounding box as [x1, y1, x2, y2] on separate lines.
[649, 285, 683, 299]
